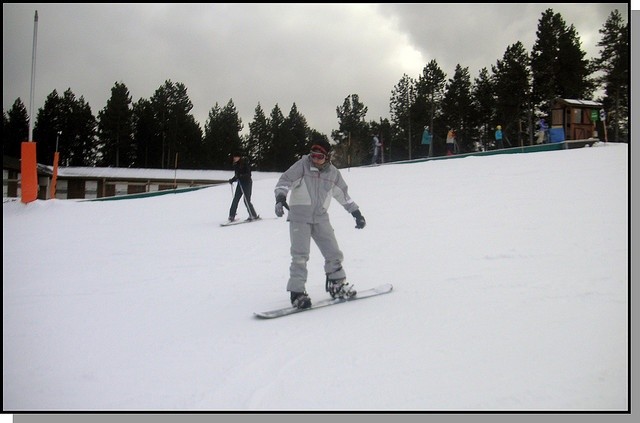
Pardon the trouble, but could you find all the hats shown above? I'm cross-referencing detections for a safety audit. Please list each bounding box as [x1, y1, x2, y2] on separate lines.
[232, 151, 242, 157]
[311, 138, 331, 155]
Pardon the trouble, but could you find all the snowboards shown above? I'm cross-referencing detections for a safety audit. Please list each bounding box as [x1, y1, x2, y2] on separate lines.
[253, 282, 393, 319]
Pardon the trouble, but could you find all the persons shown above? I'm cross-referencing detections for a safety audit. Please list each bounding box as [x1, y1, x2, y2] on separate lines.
[226, 151, 258, 222]
[495, 126, 504, 148]
[446, 127, 454, 154]
[537, 118, 550, 144]
[422, 125, 433, 157]
[371, 134, 382, 165]
[274, 139, 366, 308]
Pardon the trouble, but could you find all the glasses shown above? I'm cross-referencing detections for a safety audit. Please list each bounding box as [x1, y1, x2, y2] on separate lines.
[310, 152, 326, 161]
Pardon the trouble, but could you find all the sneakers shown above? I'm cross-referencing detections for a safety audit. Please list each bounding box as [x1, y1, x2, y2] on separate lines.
[330, 282, 356, 300]
[290, 291, 312, 309]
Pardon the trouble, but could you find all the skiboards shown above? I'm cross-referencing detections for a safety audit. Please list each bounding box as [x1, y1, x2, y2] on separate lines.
[221, 213, 261, 226]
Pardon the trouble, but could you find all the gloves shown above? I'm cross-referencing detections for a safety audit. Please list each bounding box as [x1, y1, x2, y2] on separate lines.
[275, 196, 290, 218]
[355, 215, 365, 229]
